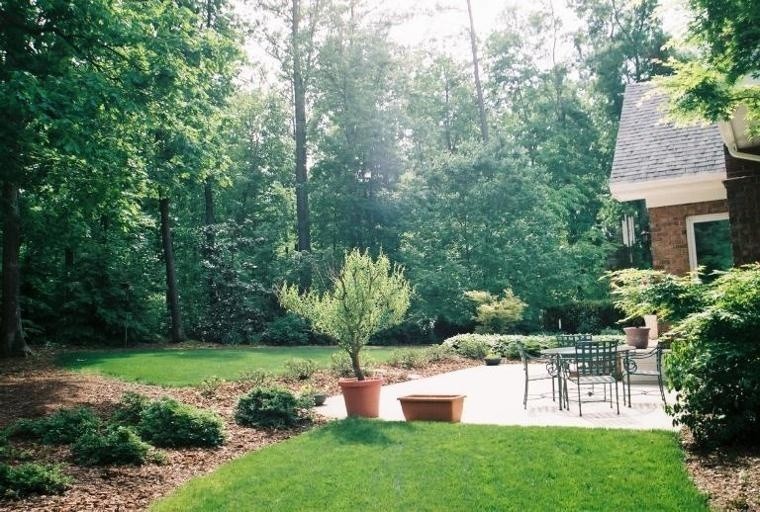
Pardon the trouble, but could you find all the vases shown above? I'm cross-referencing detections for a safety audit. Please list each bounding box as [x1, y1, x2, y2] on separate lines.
[624, 326, 651, 350]
[397, 394, 465, 423]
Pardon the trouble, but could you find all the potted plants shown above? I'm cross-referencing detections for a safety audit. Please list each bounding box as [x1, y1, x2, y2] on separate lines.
[272, 242, 424, 421]
[484, 354, 501, 365]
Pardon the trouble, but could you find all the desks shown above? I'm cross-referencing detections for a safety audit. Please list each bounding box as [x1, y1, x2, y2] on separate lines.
[540, 346, 635, 407]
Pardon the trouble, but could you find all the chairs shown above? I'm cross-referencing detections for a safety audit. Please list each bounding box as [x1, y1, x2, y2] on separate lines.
[516, 333, 620, 416]
